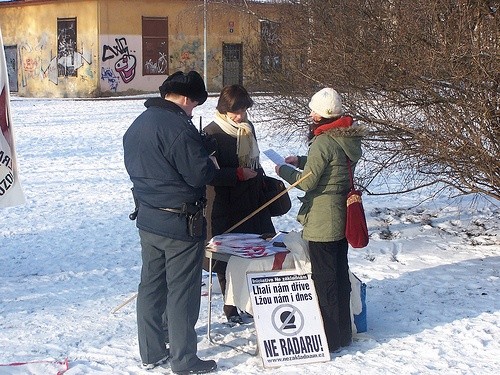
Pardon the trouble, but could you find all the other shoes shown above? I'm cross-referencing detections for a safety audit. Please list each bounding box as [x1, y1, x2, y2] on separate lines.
[171, 355, 217, 375]
[223, 304, 242, 324]
[140, 348, 170, 370]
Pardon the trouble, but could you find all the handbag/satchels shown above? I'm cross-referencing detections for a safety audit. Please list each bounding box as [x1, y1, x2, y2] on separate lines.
[344, 189, 369, 249]
[350, 270, 367, 333]
[260, 167, 292, 217]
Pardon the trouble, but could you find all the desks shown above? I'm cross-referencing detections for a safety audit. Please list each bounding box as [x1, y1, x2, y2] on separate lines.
[205, 251, 258, 356]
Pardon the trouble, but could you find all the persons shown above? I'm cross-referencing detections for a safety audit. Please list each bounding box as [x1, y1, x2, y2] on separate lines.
[122, 72, 217, 375]
[202, 85, 276, 322]
[275, 88, 366, 353]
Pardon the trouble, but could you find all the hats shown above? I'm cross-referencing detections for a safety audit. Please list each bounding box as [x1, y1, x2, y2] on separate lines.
[309, 87, 345, 118]
[159, 70, 208, 106]
